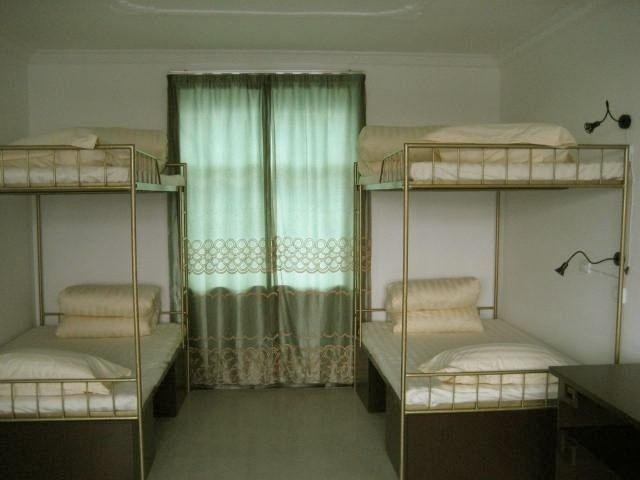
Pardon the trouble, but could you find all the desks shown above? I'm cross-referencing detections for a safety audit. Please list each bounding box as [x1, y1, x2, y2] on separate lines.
[546, 361, 640, 480]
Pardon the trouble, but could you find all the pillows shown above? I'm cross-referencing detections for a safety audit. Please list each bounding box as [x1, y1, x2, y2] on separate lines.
[0, 346, 132, 397]
[0, 127, 115, 168]
[419, 122, 578, 165]
[417, 343, 571, 385]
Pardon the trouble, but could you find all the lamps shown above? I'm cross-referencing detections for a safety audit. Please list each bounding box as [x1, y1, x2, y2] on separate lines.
[584, 99, 631, 134]
[554, 250, 626, 277]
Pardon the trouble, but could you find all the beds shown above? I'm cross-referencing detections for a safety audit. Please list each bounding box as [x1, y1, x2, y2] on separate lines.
[350, 140, 632, 480]
[1, 143, 186, 480]
